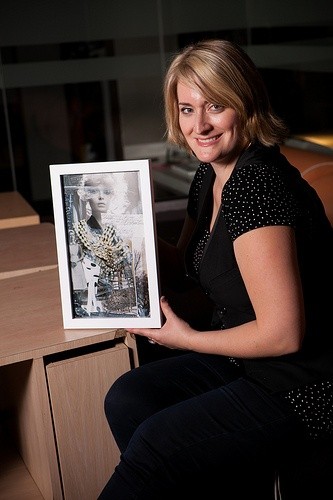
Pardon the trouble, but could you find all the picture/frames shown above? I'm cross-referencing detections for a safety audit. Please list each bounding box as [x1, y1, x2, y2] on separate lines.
[49, 158, 164, 330]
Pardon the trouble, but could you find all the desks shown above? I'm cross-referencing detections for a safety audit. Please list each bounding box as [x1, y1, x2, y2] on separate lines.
[0, 190, 41, 231]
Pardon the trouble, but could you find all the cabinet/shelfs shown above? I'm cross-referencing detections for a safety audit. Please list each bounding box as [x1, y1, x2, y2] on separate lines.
[0, 223, 141, 500]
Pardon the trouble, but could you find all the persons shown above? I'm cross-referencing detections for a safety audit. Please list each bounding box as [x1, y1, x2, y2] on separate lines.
[68, 172, 150, 318]
[96, 41, 332, 500]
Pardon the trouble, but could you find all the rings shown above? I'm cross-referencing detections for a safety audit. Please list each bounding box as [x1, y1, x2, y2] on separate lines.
[148, 339, 155, 345]
